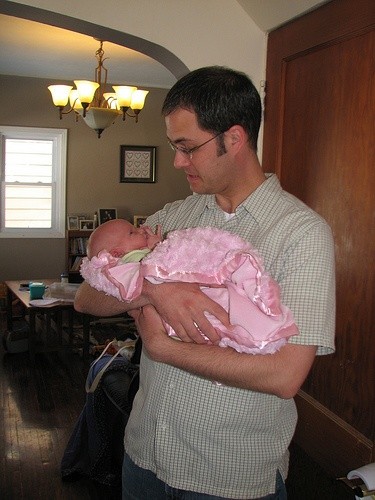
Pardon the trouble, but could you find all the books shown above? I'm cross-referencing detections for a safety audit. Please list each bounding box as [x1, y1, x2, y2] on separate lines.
[68, 236, 90, 255]
[70, 256, 83, 271]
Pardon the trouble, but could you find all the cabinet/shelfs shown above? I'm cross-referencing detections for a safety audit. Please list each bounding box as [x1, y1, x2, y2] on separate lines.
[64, 229, 94, 283]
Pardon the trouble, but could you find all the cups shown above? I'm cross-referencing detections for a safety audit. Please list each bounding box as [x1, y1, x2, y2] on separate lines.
[29, 281, 48, 300]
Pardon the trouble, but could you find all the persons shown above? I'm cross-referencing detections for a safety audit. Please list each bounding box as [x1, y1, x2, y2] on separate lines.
[80, 219, 300, 356]
[101, 211, 113, 223]
[83, 223, 93, 229]
[74, 66, 337, 500]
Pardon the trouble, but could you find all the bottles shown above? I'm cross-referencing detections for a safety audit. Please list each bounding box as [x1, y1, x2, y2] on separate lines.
[61, 275, 68, 283]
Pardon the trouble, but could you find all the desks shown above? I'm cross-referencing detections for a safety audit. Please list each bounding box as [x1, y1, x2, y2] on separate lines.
[3, 278, 90, 372]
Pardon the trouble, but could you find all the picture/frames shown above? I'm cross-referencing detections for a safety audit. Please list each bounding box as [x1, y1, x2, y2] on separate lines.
[133, 215, 148, 228]
[120, 146, 157, 184]
[68, 213, 95, 231]
[98, 207, 117, 226]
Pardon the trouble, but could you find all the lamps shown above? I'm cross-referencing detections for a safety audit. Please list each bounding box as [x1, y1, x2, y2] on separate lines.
[48, 38, 149, 140]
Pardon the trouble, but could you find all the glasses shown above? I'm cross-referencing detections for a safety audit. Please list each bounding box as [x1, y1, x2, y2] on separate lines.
[167, 124, 237, 155]
